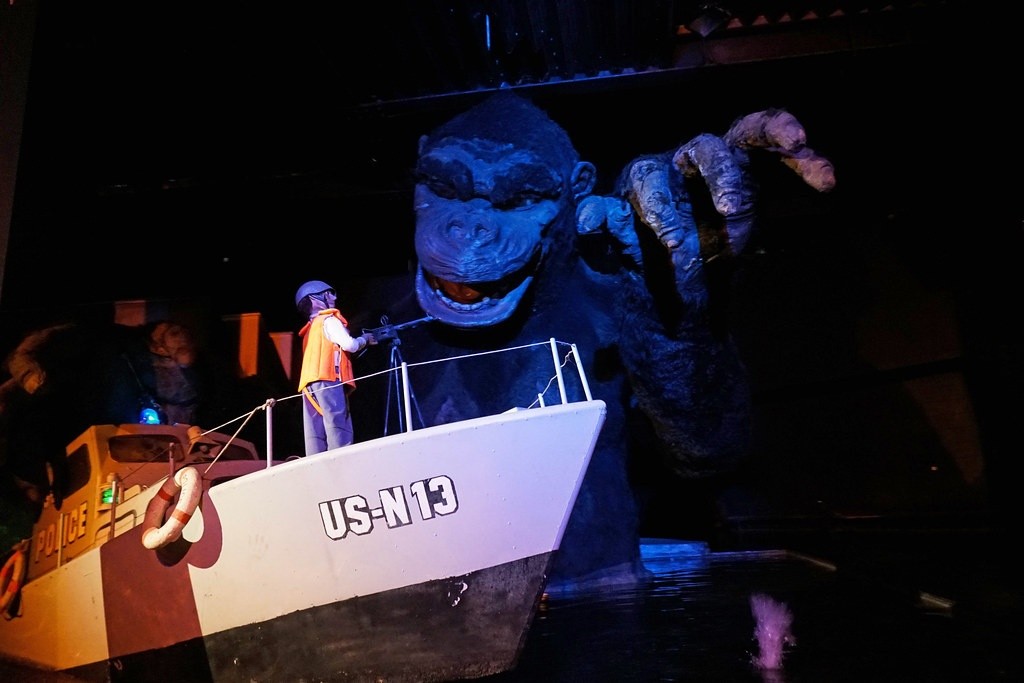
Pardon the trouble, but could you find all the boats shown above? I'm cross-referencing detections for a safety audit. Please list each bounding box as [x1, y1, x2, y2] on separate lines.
[0, 337, 609, 683]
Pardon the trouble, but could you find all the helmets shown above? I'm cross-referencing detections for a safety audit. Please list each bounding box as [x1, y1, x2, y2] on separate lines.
[296, 280, 335, 305]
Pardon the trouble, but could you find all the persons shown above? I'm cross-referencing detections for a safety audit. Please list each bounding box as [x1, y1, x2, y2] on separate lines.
[295, 280, 378, 457]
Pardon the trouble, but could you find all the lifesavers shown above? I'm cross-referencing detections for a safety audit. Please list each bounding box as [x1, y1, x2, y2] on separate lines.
[140, 465, 202, 552]
[0, 551, 27, 610]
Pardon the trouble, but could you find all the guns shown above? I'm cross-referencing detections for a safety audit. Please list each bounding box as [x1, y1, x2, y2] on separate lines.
[361, 310, 440, 345]
